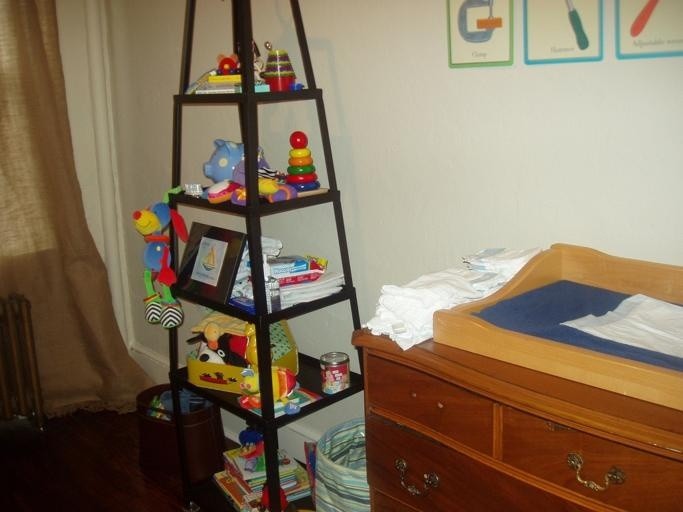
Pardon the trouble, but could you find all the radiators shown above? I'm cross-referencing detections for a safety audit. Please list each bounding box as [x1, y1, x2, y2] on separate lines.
[0, 294, 48, 450]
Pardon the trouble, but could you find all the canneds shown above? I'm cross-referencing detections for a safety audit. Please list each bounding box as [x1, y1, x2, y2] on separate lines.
[320, 351, 351, 395]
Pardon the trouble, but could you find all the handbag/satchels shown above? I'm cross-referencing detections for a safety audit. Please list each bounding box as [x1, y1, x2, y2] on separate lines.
[134, 381, 228, 500]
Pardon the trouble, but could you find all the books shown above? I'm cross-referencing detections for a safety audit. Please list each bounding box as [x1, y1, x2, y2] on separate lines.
[211, 444, 312, 511]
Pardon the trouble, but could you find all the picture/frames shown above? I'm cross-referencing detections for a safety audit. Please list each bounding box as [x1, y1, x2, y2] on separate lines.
[177, 222, 247, 305]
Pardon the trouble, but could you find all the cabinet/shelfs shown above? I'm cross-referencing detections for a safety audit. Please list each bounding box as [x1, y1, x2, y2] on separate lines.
[352, 330, 683, 512]
[169, 0, 364, 512]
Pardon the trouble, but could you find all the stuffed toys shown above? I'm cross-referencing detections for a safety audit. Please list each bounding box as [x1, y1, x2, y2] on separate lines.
[190, 310, 247, 351]
[200, 139, 244, 200]
[131, 186, 190, 330]
[231, 159, 297, 207]
[207, 179, 241, 207]
[186, 333, 246, 368]
[238, 365, 299, 409]
